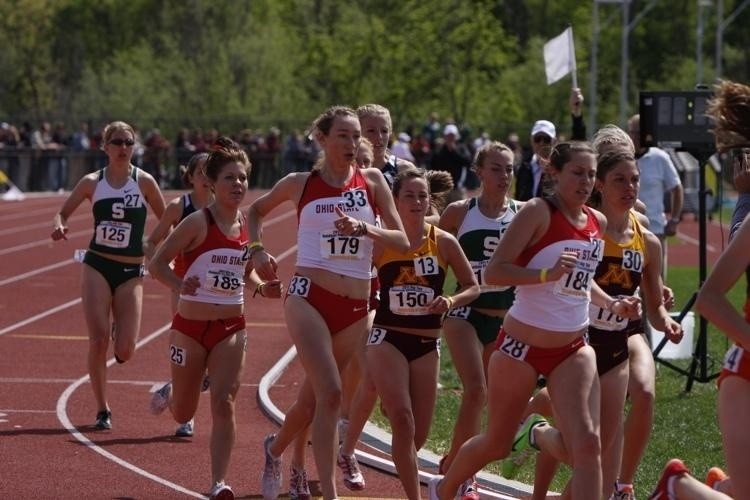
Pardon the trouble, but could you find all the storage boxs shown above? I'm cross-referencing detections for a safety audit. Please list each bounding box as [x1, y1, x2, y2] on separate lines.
[645, 311, 695, 359]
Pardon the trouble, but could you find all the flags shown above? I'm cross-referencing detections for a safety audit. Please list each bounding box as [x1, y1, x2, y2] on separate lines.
[543, 26, 577, 86]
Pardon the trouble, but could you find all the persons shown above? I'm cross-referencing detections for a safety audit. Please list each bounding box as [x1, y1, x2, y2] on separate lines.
[51, 120, 167, 430]
[141, 81, 749, 499]
[1, 117, 109, 192]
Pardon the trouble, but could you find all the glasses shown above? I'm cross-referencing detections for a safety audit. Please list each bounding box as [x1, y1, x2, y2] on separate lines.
[534, 135, 551, 143]
[107, 138, 134, 147]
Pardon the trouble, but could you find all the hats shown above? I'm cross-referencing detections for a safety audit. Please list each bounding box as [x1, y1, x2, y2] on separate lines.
[444, 124, 460, 140]
[531, 120, 557, 140]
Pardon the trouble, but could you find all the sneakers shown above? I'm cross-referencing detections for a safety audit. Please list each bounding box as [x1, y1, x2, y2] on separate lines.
[427, 454, 480, 500]
[111, 321, 125, 363]
[175, 416, 194, 437]
[337, 418, 365, 491]
[150, 380, 173, 416]
[499, 412, 549, 479]
[261, 432, 313, 500]
[209, 477, 235, 500]
[94, 410, 112, 429]
[609, 458, 730, 500]
